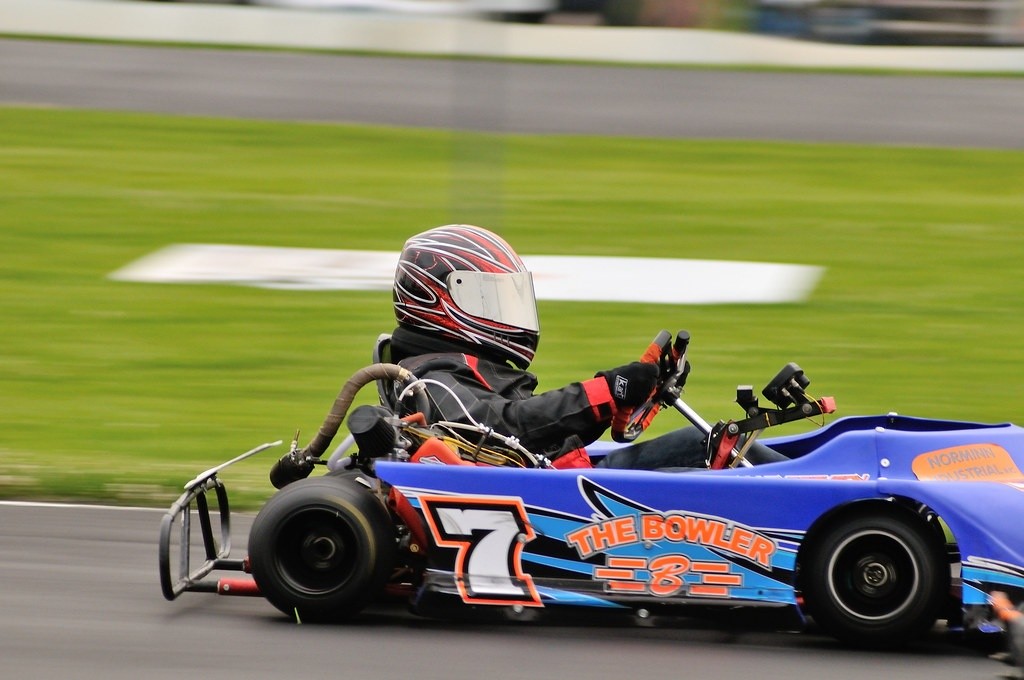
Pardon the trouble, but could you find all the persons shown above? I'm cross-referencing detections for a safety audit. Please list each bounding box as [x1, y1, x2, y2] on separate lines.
[387, 224, 793, 474]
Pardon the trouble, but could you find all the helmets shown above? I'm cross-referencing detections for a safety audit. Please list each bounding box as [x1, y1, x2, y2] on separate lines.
[393, 223, 540, 373]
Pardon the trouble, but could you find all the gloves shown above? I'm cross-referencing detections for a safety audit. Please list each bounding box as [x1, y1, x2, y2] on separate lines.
[594, 360, 661, 411]
